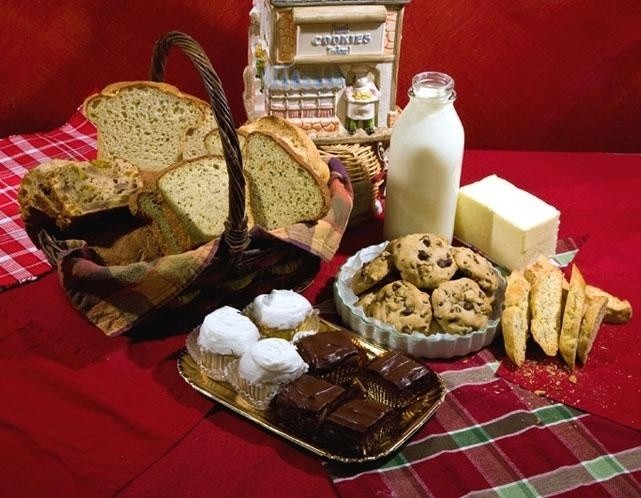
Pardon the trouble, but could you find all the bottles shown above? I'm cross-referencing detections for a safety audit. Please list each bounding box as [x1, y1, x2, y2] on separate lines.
[382, 71, 468, 244]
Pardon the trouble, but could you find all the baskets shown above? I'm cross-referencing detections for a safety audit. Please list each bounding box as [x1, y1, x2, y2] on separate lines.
[318, 142, 383, 223]
[39, 31, 342, 338]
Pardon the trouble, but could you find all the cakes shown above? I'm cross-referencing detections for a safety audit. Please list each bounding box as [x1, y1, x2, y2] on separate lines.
[244, 0, 409, 137]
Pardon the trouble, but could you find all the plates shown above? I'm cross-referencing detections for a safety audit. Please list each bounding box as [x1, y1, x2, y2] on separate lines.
[334, 237, 512, 360]
[176, 297, 445, 461]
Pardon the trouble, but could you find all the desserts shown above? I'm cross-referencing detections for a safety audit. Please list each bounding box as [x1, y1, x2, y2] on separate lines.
[196, 288, 442, 456]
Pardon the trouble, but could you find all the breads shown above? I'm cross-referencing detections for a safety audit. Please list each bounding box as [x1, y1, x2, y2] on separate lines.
[20, 81, 328, 265]
[502, 258, 632, 365]
[454, 175, 561, 271]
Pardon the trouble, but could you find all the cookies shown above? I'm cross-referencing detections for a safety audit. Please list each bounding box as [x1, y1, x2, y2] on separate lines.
[350, 232, 500, 334]
